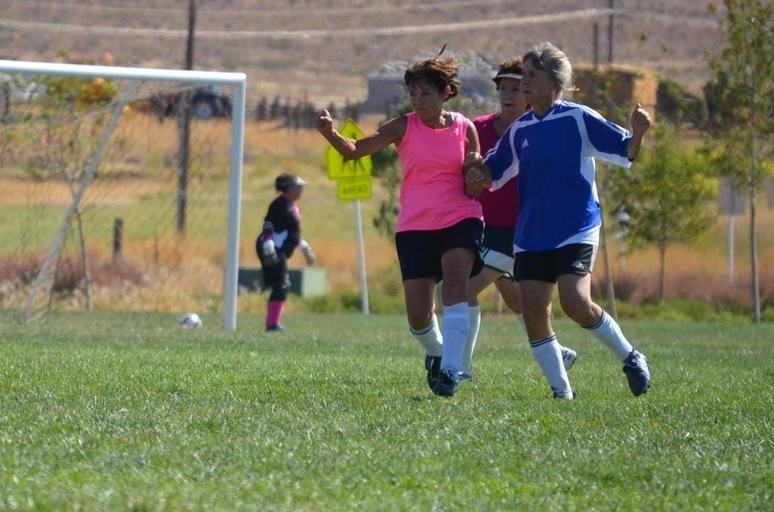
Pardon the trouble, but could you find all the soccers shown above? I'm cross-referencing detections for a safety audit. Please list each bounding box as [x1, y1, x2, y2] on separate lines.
[179, 313, 201, 330]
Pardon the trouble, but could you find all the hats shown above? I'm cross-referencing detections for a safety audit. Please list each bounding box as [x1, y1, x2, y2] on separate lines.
[279, 176, 306, 188]
[493, 68, 523, 83]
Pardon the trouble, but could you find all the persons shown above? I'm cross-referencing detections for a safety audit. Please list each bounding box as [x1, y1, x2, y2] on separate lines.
[450, 58, 573, 378]
[313, 42, 482, 398]
[465, 42, 652, 402]
[256, 174, 315, 332]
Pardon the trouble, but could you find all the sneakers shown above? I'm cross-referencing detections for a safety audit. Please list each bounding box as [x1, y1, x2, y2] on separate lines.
[266, 325, 285, 331]
[456, 370, 472, 382]
[439, 368, 456, 397]
[562, 347, 577, 371]
[623, 347, 650, 397]
[425, 356, 442, 391]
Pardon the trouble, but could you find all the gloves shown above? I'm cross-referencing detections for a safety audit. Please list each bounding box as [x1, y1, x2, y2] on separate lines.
[262, 240, 279, 267]
[299, 241, 316, 266]
